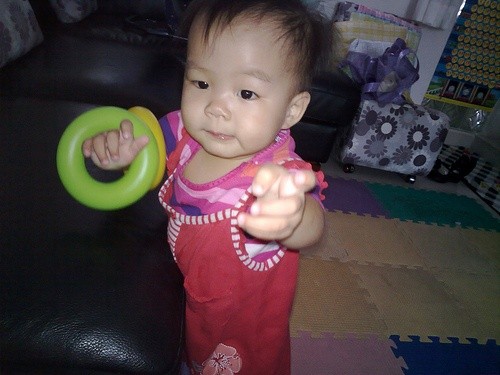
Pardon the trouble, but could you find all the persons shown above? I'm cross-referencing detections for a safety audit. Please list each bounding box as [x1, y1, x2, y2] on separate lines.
[81, 0, 345, 375]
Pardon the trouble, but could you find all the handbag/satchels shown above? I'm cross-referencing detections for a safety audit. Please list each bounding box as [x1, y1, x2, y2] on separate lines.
[340, 37, 421, 107]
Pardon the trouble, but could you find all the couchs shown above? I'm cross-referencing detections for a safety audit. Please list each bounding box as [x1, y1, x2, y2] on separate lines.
[0, 0, 364, 375]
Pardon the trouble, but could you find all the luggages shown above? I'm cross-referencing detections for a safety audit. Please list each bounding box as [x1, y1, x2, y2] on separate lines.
[335, 92, 450, 184]
